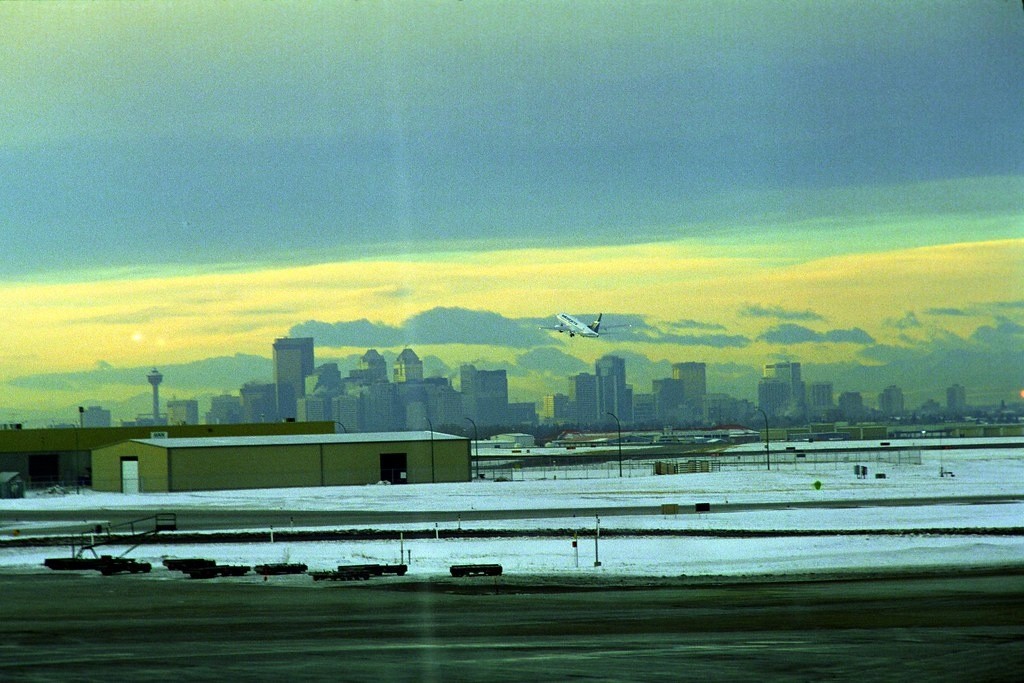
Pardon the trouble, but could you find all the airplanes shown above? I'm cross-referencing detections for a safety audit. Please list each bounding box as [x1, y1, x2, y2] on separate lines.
[538, 312, 603, 338]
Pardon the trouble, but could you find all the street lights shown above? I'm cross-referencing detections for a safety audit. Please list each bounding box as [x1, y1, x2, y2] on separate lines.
[466, 418, 478, 478]
[71, 424, 79, 494]
[755, 408, 770, 470]
[607, 412, 622, 477]
